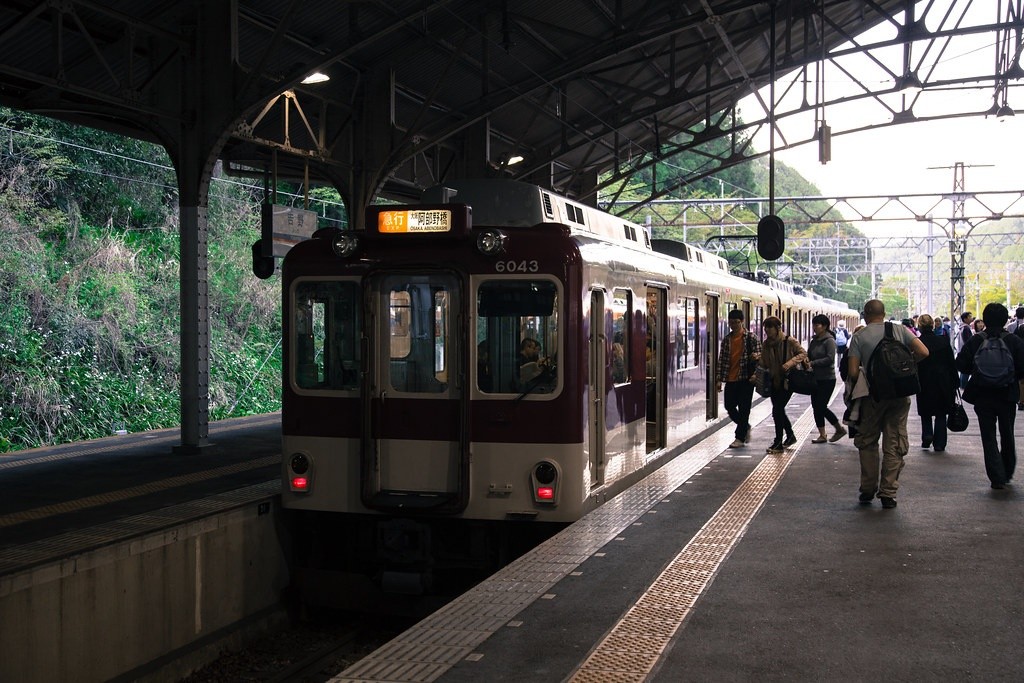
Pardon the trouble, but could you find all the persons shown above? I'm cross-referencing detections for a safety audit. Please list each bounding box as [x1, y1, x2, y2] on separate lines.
[1005, 306, 1024, 410]
[751, 316, 807, 452]
[889, 311, 1016, 389]
[716, 309, 763, 447]
[834, 319, 851, 367]
[849, 299, 929, 509]
[478, 332, 656, 393]
[956, 303, 1024, 489]
[839, 325, 865, 438]
[807, 314, 847, 443]
[912, 314, 961, 452]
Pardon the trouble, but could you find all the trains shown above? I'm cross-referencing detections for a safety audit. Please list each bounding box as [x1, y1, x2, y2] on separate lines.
[278, 172, 862, 525]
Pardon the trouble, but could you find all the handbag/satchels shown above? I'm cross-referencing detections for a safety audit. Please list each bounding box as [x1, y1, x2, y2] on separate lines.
[755, 367, 770, 397]
[793, 362, 813, 395]
[947, 388, 968, 431]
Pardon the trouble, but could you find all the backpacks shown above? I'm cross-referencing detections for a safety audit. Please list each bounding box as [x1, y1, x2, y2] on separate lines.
[866, 321, 920, 403]
[971, 332, 1015, 393]
[835, 328, 847, 346]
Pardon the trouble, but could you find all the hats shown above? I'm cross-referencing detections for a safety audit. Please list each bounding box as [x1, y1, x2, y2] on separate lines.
[837, 319, 846, 328]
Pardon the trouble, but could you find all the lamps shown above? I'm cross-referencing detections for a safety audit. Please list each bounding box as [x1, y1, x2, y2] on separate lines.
[889, 0, 1024, 125]
[288, 63, 331, 85]
[499, 151, 524, 165]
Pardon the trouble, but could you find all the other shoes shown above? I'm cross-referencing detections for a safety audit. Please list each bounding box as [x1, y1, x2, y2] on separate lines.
[783, 435, 798, 448]
[729, 438, 744, 448]
[766, 440, 784, 453]
[743, 426, 752, 443]
[882, 498, 897, 509]
[829, 429, 847, 442]
[1018, 405, 1024, 409]
[921, 445, 929, 450]
[811, 435, 827, 443]
[991, 484, 1002, 489]
[859, 492, 874, 501]
